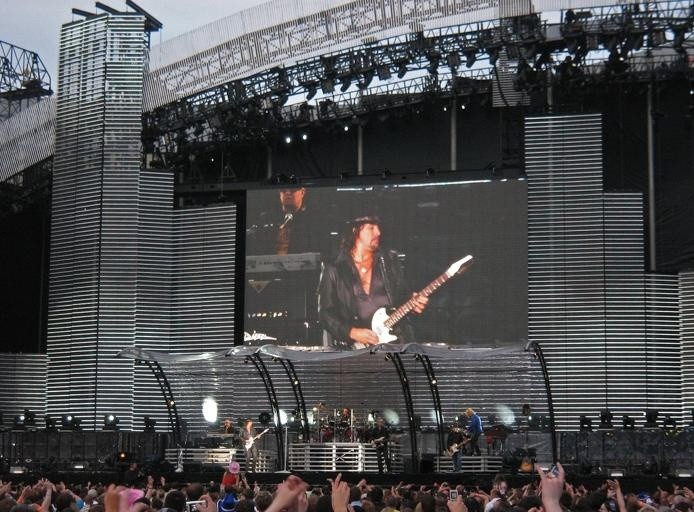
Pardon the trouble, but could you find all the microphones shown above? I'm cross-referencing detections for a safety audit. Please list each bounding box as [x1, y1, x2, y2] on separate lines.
[280, 213, 293, 229]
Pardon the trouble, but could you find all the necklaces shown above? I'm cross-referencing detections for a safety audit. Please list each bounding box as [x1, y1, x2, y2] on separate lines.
[360, 266, 368, 273]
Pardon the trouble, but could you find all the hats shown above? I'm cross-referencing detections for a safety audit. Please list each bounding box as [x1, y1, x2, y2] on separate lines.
[345, 200, 383, 223]
[229, 462, 241, 474]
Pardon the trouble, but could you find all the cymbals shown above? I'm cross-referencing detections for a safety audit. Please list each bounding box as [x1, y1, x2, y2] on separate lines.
[313, 402, 328, 410]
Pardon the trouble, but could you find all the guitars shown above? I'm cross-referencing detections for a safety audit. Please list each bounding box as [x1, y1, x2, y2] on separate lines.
[447, 438, 472, 456]
[371, 436, 400, 448]
[521, 443, 534, 473]
[332, 254, 475, 352]
[242, 428, 269, 452]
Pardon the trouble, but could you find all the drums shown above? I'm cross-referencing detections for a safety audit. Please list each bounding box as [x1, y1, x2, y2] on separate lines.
[320, 425, 337, 442]
[344, 426, 360, 442]
[329, 415, 340, 427]
[341, 415, 351, 427]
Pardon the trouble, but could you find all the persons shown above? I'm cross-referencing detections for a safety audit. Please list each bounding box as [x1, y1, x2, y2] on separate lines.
[369, 413, 393, 475]
[340, 407, 351, 422]
[251, 189, 333, 344]
[1, 458, 694, 511]
[447, 413, 469, 473]
[238, 417, 261, 474]
[221, 419, 237, 447]
[314, 206, 430, 344]
[463, 408, 484, 457]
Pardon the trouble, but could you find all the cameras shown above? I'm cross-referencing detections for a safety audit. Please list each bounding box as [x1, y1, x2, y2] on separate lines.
[449, 490, 458, 502]
[186, 500, 207, 512]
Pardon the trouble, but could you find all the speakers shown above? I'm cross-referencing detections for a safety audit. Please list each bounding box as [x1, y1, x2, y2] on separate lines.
[402, 453, 414, 474]
[418, 453, 435, 473]
